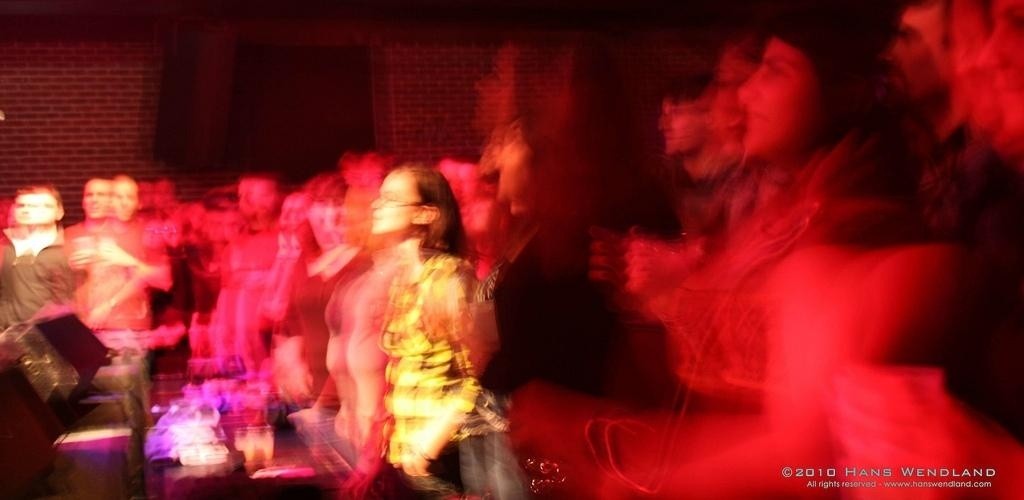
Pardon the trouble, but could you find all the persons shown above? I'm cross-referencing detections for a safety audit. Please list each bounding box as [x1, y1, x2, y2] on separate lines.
[0, 1, 1024, 499]
